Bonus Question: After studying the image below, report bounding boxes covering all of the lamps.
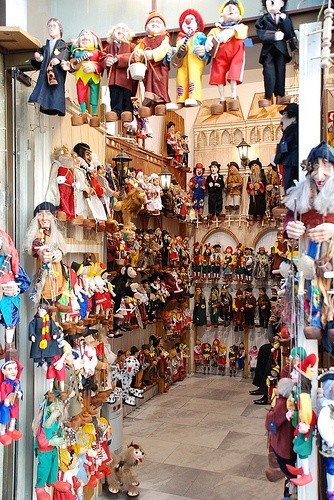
[112,149,133,200]
[158,165,173,217]
[237,137,251,168]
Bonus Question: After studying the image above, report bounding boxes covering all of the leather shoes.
[249,387,263,395]
[253,395,268,405]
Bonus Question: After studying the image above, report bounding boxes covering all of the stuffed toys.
[0,1,334,500]
